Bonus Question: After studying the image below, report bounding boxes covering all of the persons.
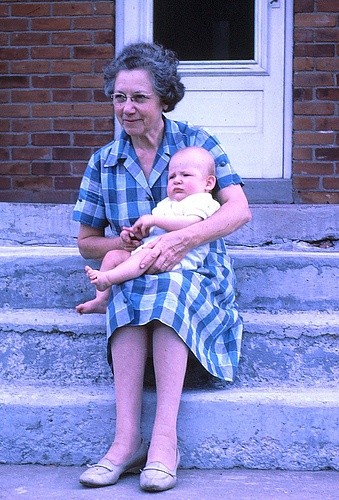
[75,145,222,317]
[69,40,255,495]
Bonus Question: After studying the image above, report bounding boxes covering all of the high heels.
[139,448,180,492]
[78,437,148,486]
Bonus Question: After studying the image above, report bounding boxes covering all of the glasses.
[110,92,156,103]
